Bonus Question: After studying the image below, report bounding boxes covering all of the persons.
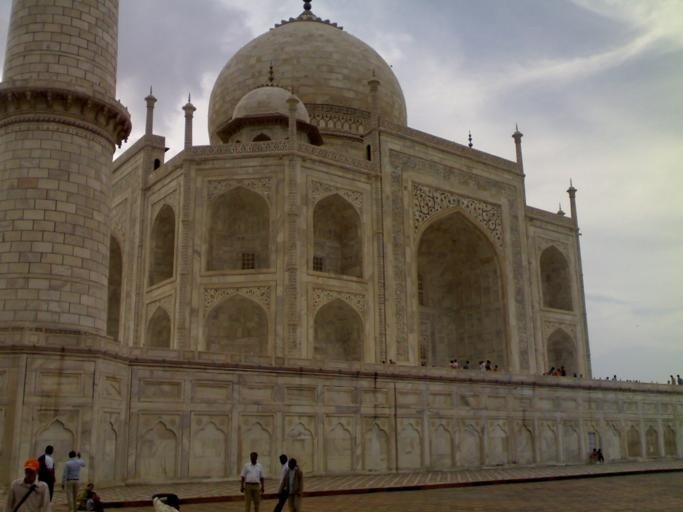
[37,445,56,503]
[667,375,681,385]
[239,452,265,512]
[607,375,617,381]
[547,366,583,378]
[272,454,289,512]
[75,483,96,512]
[151,493,181,512]
[590,448,598,462]
[450,360,499,371]
[597,448,604,464]
[85,492,104,512]
[382,360,396,364]
[60,449,86,512]
[5,458,51,512]
[279,457,304,512]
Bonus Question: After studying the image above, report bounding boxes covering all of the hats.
[23,458,40,471]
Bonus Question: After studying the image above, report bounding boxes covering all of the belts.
[246,482,259,485]
[69,479,79,480]
